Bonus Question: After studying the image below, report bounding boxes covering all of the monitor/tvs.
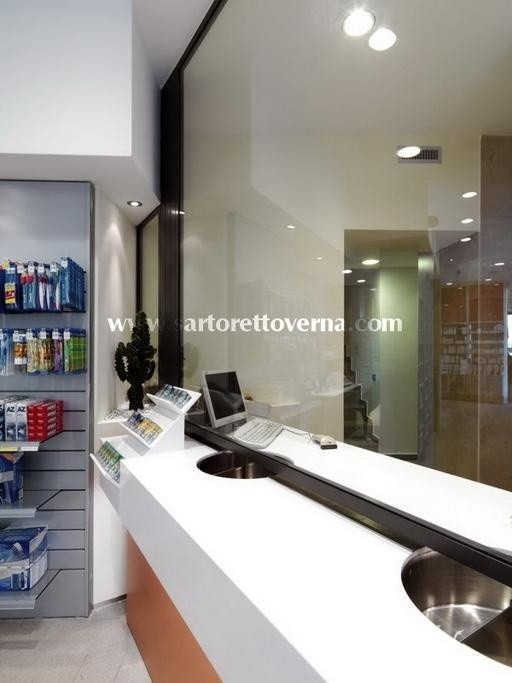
[199,368,248,437]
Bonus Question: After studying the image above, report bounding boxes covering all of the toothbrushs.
[1,259,85,375]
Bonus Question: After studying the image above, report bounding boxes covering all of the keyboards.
[234,416,284,449]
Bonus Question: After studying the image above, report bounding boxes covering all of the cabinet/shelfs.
[0,175,103,619]
[87,381,203,488]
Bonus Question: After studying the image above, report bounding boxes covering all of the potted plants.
[113,306,159,413]
